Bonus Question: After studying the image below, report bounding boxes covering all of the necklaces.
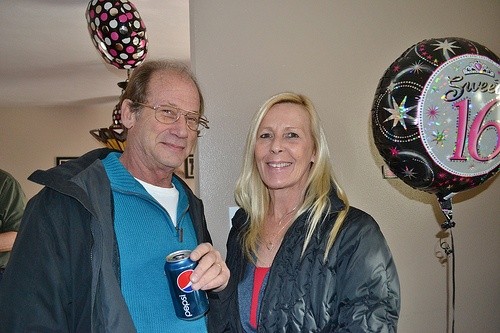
[265,217,293,251]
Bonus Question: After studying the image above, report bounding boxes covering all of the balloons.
[370,37,500,222]
[83,0,149,70]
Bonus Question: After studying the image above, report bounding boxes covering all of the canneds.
[164,249,210,321]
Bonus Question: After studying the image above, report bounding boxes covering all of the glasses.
[135,101,209,131]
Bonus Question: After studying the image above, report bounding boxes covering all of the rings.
[216,263,222,269]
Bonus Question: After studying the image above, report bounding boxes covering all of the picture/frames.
[184,154,194,178]
[56,157,80,166]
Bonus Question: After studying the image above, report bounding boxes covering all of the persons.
[226,92,401,333]
[0,57,238,333]
[0,168,30,272]
